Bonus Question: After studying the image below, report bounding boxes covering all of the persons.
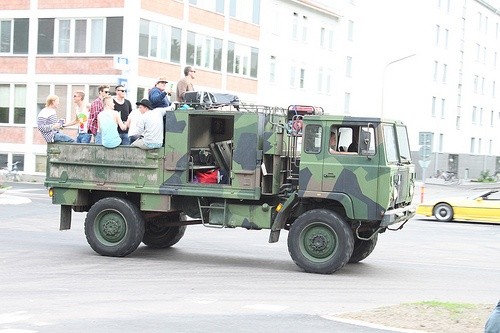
[87,84,111,144]
[127,98,179,150]
[60,91,92,143]
[113,85,133,145]
[97,97,128,149]
[148,78,171,147]
[176,65,196,102]
[329,132,359,155]
[37,95,75,143]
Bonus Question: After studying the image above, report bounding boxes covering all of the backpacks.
[199,149,215,166]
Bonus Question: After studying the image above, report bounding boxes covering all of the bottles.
[170,83,176,101]
[184,100,187,105]
[190,99,193,106]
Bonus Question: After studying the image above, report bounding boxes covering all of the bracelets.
[63,124,65,127]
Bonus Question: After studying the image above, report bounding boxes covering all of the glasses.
[74,95,80,97]
[105,91,110,94]
[190,70,196,73]
[118,90,127,92]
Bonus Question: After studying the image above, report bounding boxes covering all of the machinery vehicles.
[43,89,417,274]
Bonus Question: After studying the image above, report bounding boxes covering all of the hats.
[136,99,154,110]
[155,77,168,84]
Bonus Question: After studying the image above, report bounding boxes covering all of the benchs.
[188,147,216,183]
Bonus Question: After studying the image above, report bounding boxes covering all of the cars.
[418,186,500,224]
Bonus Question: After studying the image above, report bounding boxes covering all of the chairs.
[340,146,345,152]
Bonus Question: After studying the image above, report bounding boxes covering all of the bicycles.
[0,161,24,183]
